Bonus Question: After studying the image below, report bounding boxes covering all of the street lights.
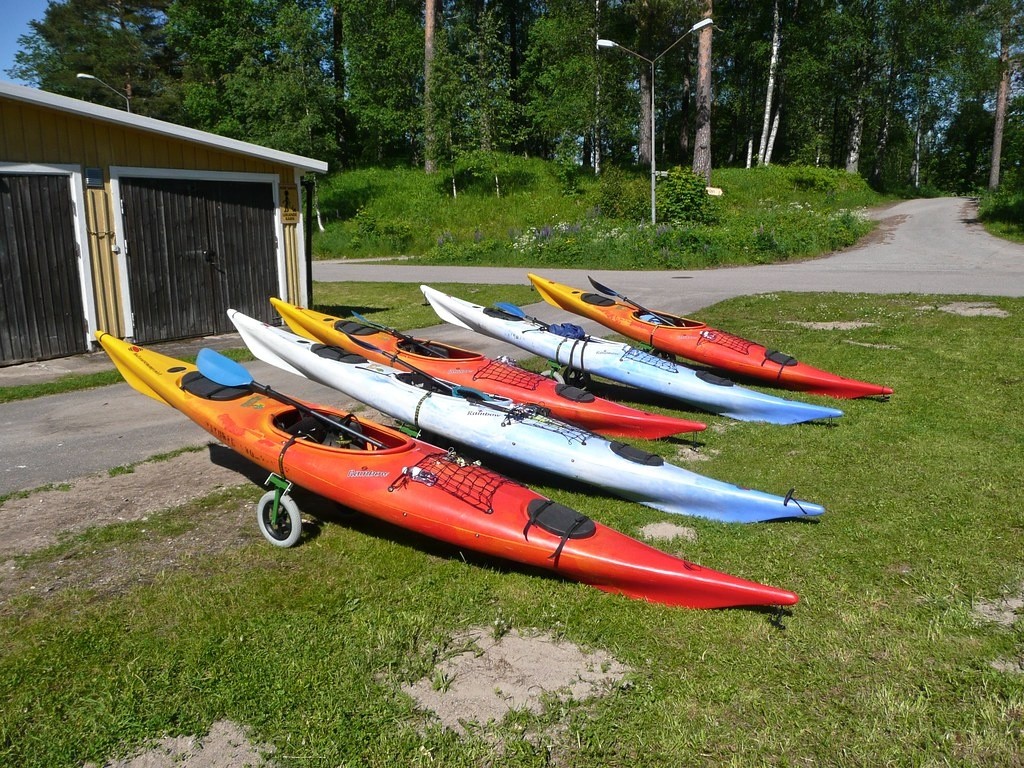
[76,72,129,113]
[597,18,713,226]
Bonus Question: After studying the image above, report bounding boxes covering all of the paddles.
[351,310,448,359]
[196,348,389,449]
[338,325,493,400]
[587,275,676,327]
[494,301,549,329]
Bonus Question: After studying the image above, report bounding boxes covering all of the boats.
[227,298,827,522]
[92,330,799,611]
[420,274,894,424]
